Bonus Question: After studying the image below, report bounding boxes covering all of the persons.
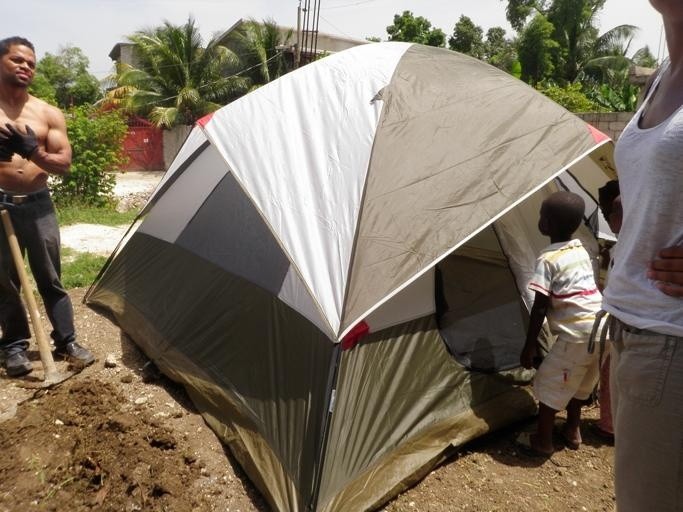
[518,180,624,455]
[0,36,95,378]
[608,0,682,512]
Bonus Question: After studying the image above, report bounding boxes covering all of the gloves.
[0,123,39,162]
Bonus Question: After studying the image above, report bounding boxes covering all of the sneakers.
[55,343,95,364]
[5,352,33,375]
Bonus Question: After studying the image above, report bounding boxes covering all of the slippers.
[553,419,578,450]
[511,431,553,457]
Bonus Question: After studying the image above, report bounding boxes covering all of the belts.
[0,189,50,206]
